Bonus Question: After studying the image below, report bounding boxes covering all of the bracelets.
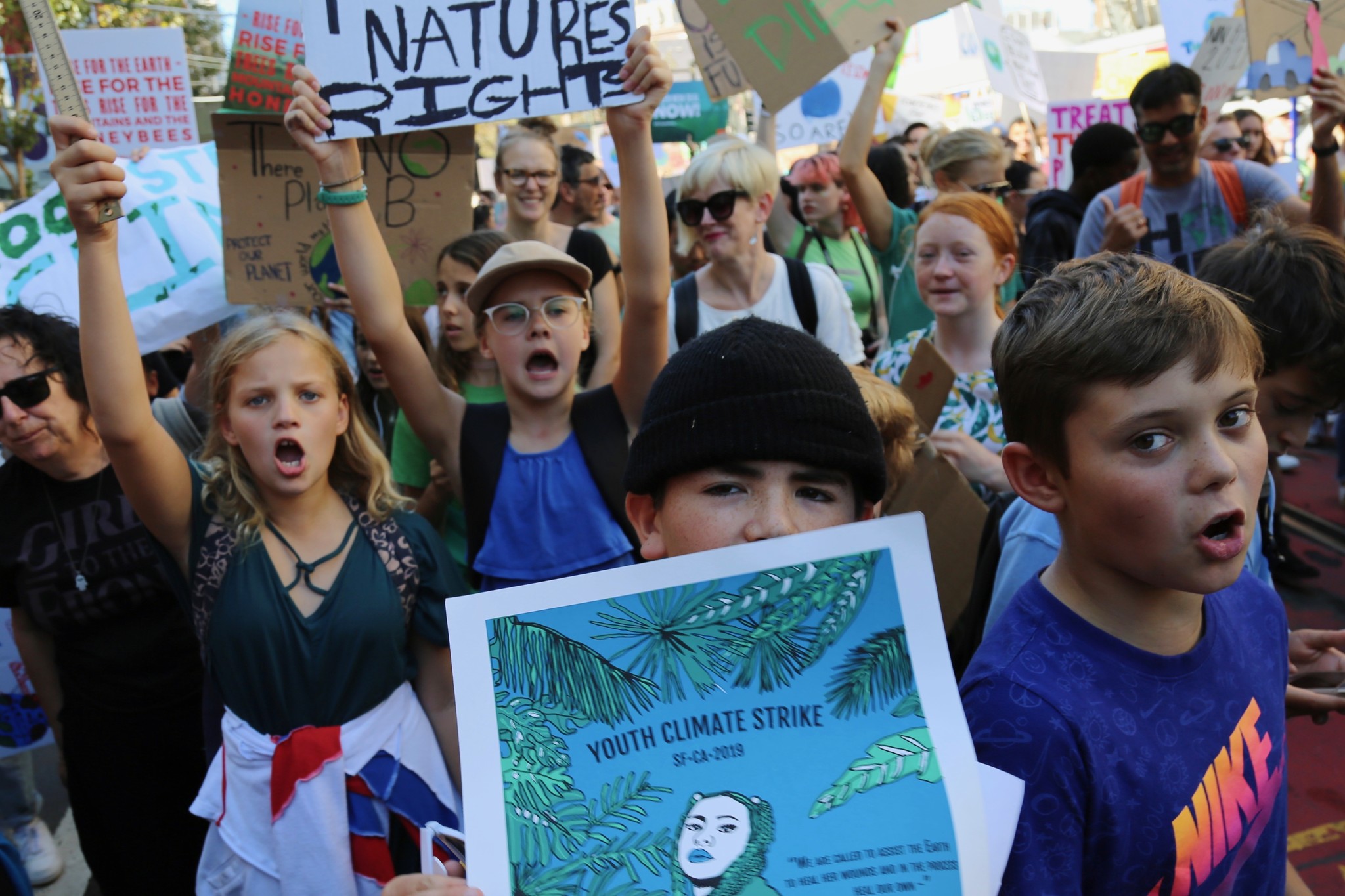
[316,170,366,187]
[316,186,369,204]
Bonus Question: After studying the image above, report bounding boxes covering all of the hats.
[623,317,890,506]
[463,239,593,315]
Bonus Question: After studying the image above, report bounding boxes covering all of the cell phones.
[1287,671,1344,696]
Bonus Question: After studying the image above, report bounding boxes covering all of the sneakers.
[12,820,64,886]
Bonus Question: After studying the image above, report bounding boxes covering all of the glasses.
[676,187,750,226]
[578,176,600,186]
[975,180,1013,196]
[1212,135,1253,153]
[0,367,59,417]
[1135,103,1200,143]
[480,296,587,337]
[502,168,559,187]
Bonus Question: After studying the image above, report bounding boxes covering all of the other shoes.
[1256,494,1323,577]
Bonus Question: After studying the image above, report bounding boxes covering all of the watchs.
[1311,134,1339,157]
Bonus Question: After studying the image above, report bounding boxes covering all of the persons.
[872,191,1022,501]
[0,19,1345,896]
[390,230,582,581]
[666,137,866,369]
[491,126,623,393]
